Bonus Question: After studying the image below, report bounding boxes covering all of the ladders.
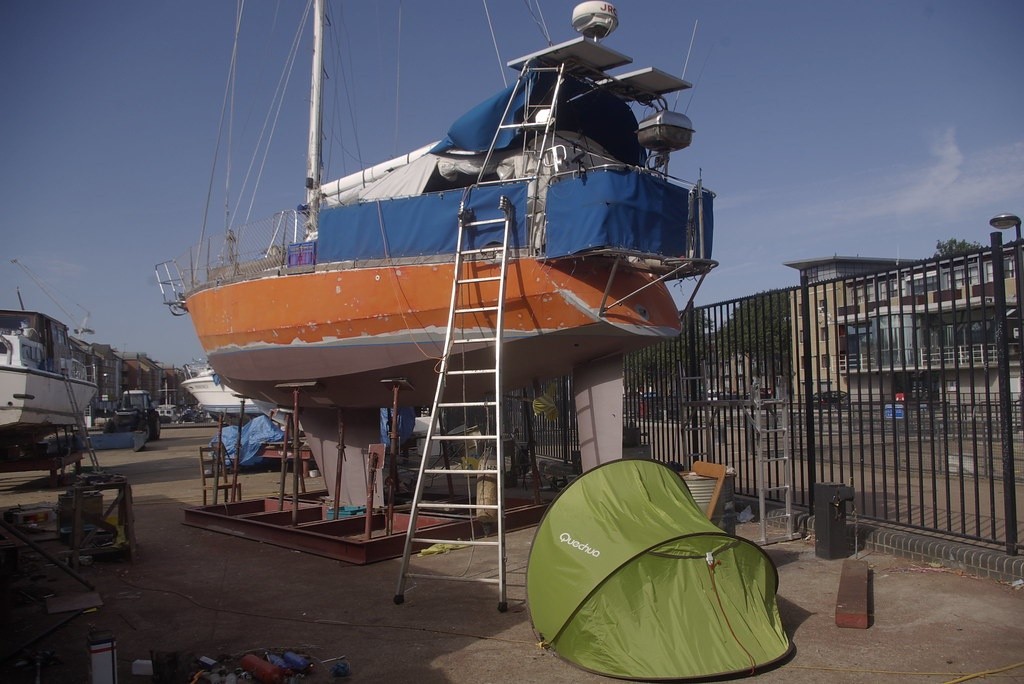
[392,195,512,614]
[61,373,101,473]
[677,360,802,546]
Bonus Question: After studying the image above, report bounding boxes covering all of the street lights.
[988,211,1024,430]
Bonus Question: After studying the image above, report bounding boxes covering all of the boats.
[0,322,101,433]
[179,353,266,416]
[148,1,722,416]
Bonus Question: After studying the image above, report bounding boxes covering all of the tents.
[524,457,793,682]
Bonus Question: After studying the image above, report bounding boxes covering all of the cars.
[812,389,849,411]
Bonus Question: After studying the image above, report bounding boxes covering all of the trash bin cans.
[814,482,848,559]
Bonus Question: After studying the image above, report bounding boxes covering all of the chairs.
[199,443,241,505]
